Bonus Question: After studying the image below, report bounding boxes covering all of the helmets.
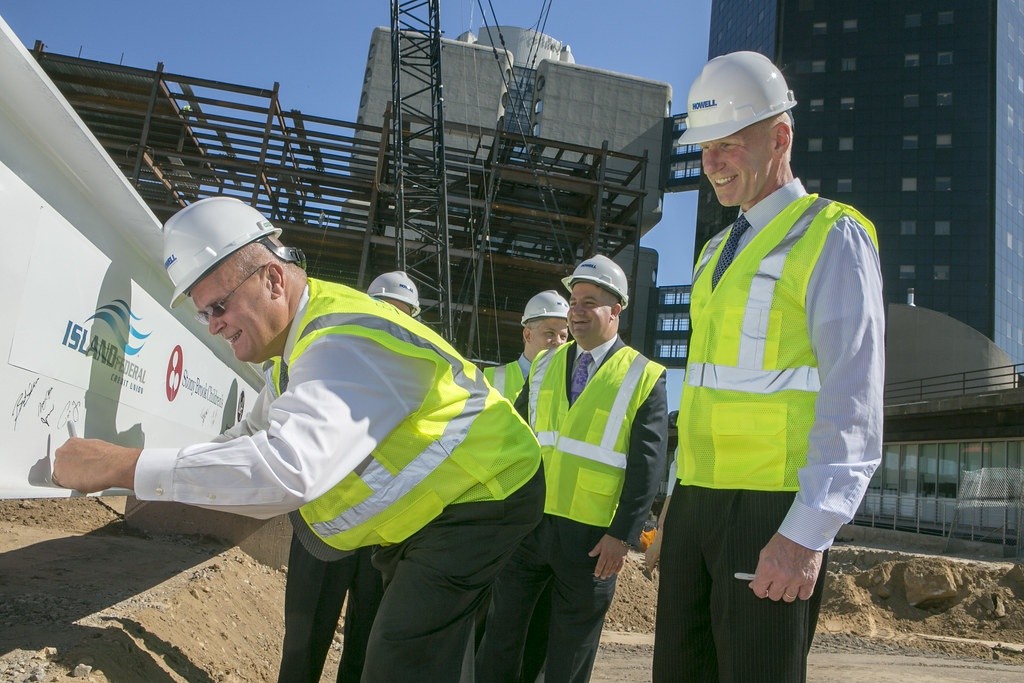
[678,51,798,145]
[561,254,629,311]
[366,270,421,318]
[162,196,306,301]
[521,290,570,326]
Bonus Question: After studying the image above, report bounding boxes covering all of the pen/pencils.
[734,572,755,581]
[67,420,77,439]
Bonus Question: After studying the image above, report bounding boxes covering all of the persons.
[475,254,669,683]
[51,197,547,682]
[479,290,569,407]
[651,50,887,683]
[277,268,421,683]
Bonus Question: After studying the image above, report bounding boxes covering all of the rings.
[785,593,797,598]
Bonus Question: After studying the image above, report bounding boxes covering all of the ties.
[571,354,593,408]
[711,211,751,292]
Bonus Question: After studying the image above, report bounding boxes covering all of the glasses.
[195,266,266,324]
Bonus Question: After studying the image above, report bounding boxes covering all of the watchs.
[621,540,632,547]
